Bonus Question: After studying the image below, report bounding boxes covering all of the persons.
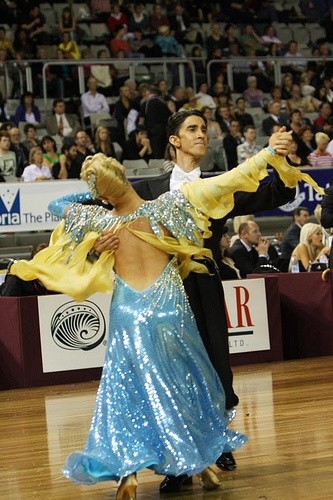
[310,193,333,271]
[51,140,81,179]
[94,126,117,159]
[230,219,277,275]
[0,0,333,181]
[237,125,264,165]
[81,75,109,125]
[58,130,93,180]
[22,123,47,155]
[0,115,324,500]
[289,223,329,274]
[45,98,81,137]
[218,226,246,279]
[9,127,31,178]
[282,208,313,264]
[72,110,307,494]
[224,117,244,172]
[15,91,42,125]
[22,146,53,180]
[89,50,117,97]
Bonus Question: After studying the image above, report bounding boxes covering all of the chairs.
[40,3,325,56]
[5,98,319,175]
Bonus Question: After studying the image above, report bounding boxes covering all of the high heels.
[199,466,222,490]
[116,471,138,500]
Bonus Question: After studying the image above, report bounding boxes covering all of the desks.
[0,271,333,391]
[0,166,333,234]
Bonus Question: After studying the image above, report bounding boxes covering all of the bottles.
[291,255,300,274]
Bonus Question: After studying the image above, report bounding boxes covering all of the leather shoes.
[160,474,192,493]
[215,452,237,471]
